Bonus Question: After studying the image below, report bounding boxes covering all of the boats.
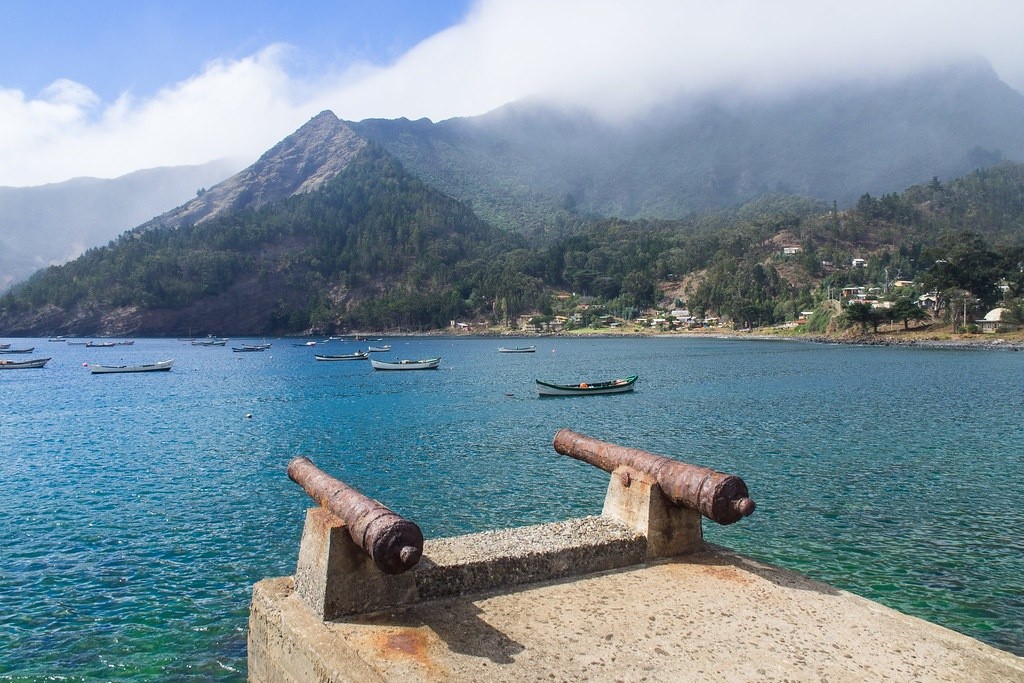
[0,347,36,354]
[48,338,66,342]
[0,343,11,349]
[314,351,370,361]
[368,345,391,353]
[109,341,135,345]
[191,333,230,347]
[0,358,52,369]
[498,344,536,354]
[536,374,639,397]
[82,358,176,374]
[231,343,274,352]
[316,336,384,346]
[177,337,197,340]
[292,341,317,347]
[371,357,441,370]
[85,342,115,347]
